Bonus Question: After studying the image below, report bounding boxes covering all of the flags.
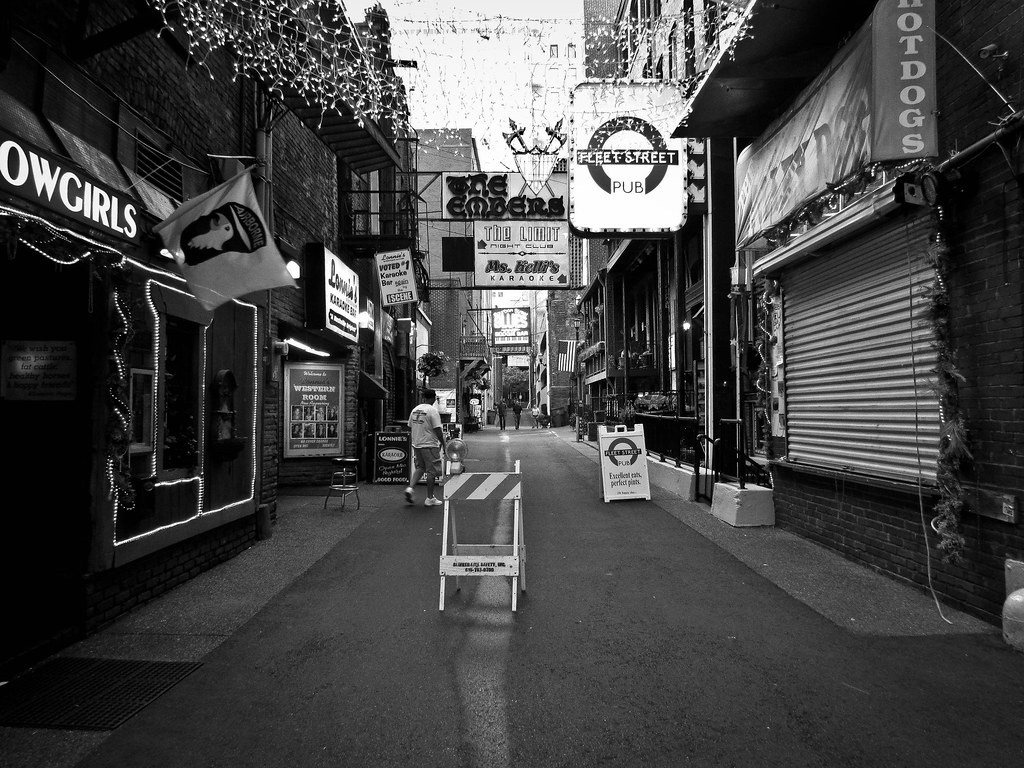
[557,340,576,373]
[156,171,296,310]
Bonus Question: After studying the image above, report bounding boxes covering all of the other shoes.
[501,428,502,430]
[515,428,518,430]
[504,427,505,430]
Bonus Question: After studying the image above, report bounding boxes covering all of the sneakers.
[424,495,443,506]
[404,486,416,504]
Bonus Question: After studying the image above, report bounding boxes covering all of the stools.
[324,457,360,512]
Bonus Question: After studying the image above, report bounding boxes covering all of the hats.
[423,389,436,398]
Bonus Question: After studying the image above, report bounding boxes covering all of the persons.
[512,401,523,430]
[404,388,447,507]
[532,404,540,429]
[497,399,507,430]
[292,406,338,438]
